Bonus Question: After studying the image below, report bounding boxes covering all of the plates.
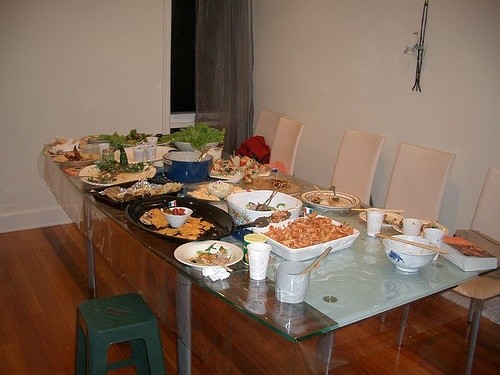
[174,240,244,268]
[266,248,357,282]
[125,195,236,244]
[44,141,305,210]
[252,215,360,262]
[300,190,450,237]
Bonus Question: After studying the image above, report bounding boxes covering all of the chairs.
[250,110,500,375]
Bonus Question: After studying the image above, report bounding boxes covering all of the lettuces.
[169,122,226,149]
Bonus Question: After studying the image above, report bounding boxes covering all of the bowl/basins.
[171,138,219,152]
[382,235,438,273]
[163,150,213,183]
[160,207,193,228]
[381,270,433,302]
[227,190,303,232]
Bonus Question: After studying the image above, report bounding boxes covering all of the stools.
[75,292,165,375]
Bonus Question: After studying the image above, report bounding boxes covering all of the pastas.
[264,211,353,249]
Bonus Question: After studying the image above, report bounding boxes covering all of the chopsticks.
[375,233,448,255]
[350,208,405,212]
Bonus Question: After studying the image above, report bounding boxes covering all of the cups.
[272,261,311,304]
[272,299,305,334]
[246,242,272,281]
[246,278,268,304]
[242,234,268,268]
[80,144,98,155]
[361,235,380,265]
[134,136,158,162]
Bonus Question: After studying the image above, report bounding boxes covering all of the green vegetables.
[90,128,168,173]
[205,242,227,255]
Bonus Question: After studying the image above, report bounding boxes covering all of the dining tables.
[37,143,500,375]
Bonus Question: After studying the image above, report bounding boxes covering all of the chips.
[146,209,213,240]
